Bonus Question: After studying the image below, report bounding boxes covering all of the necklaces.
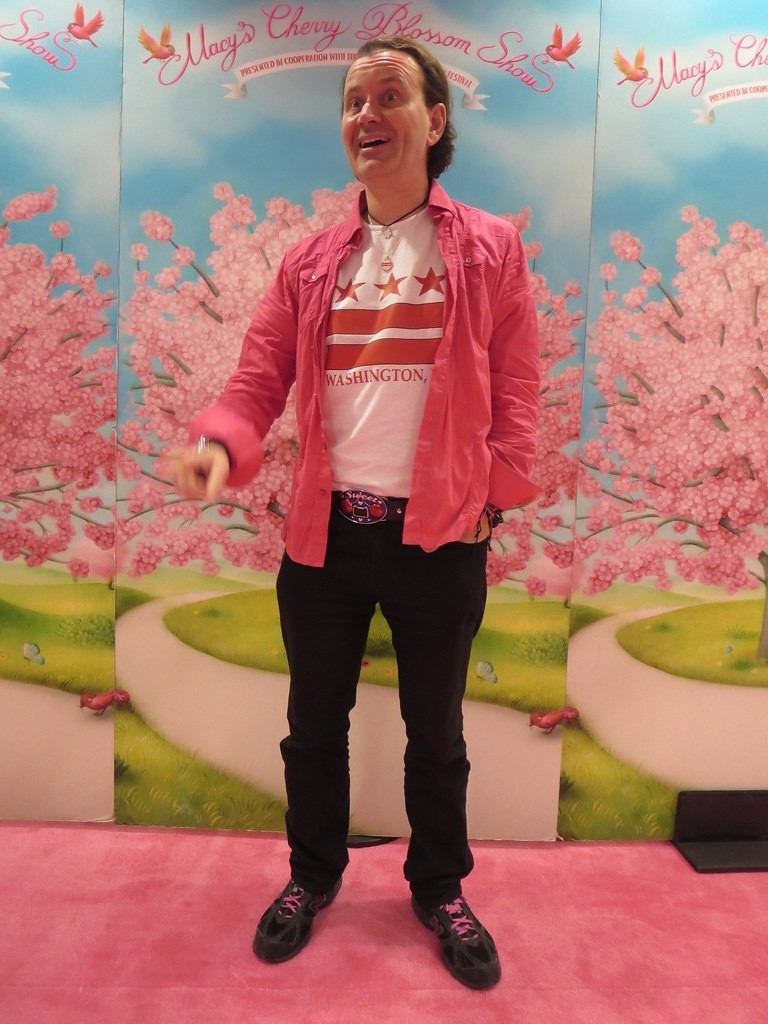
[368,198,428,238]
[368,213,418,270]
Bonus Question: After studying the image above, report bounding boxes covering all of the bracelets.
[197,435,231,466]
[484,503,503,527]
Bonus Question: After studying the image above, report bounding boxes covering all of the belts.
[330,488,407,526]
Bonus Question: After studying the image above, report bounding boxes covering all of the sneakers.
[411,894,500,991]
[253,872,343,964]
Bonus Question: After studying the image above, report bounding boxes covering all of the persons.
[174,37,539,991]
